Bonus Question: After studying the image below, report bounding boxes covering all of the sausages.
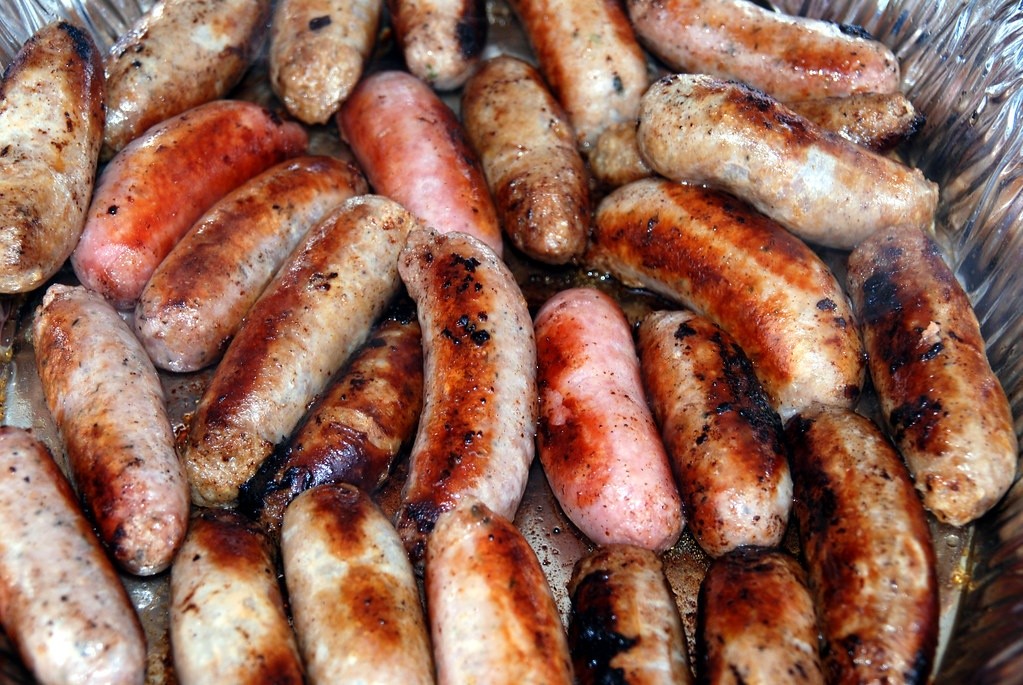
[0,0,1023,685]
